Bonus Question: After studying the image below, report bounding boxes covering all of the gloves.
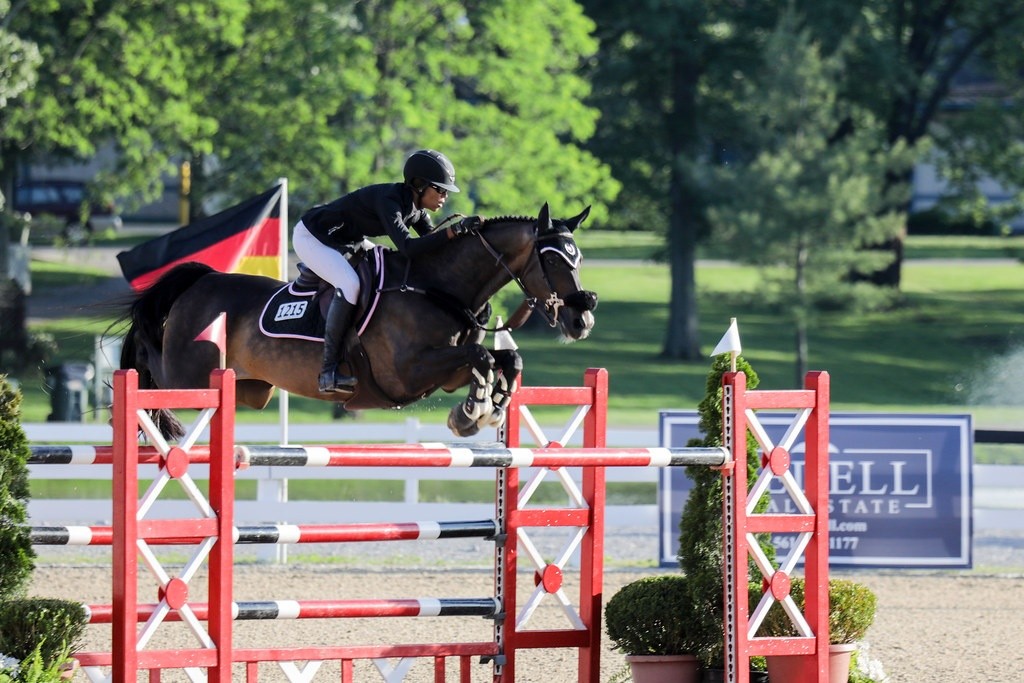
[451,216,481,236]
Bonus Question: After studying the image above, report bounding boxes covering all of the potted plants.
[679,347,780,683]
[606,577,722,682]
[742,579,876,683]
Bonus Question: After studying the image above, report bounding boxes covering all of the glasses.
[428,183,447,194]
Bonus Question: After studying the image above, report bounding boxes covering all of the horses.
[106,199,598,445]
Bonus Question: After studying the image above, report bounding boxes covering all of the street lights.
[789,112,861,394]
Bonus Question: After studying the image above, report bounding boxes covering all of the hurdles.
[18,366,833,682]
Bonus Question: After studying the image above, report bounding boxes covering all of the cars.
[10,177,125,248]
[915,160,1024,242]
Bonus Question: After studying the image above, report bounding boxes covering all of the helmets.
[404,149,460,193]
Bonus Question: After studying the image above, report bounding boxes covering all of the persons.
[292,148,460,395]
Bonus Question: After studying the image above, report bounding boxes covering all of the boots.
[317,288,358,392]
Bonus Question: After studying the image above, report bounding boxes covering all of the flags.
[116,183,281,289]
